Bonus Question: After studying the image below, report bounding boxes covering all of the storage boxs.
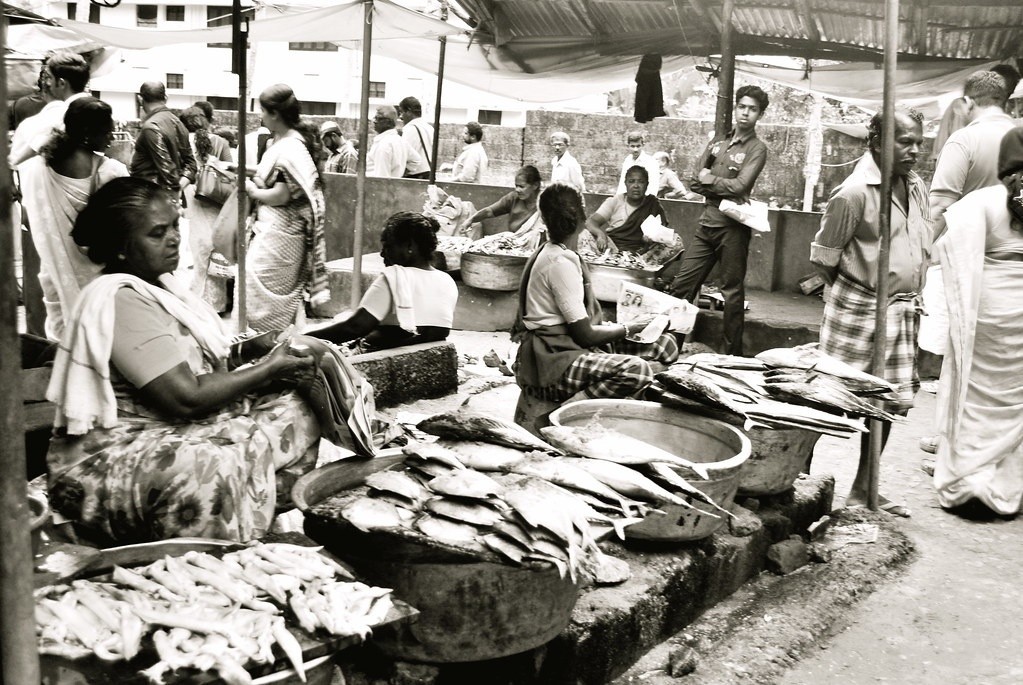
[798,272,824,296]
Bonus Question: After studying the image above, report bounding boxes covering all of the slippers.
[846,499,907,517]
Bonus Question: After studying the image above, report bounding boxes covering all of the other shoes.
[920,434,938,453]
[920,458,935,475]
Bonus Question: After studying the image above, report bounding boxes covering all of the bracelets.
[228,347,237,368]
[619,322,629,339]
[237,343,244,364]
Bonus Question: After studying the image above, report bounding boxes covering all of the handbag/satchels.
[193,135,236,205]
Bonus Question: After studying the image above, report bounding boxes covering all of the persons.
[299,209,460,352]
[47,175,328,545]
[621,292,642,309]
[668,85,770,356]
[5,47,434,346]
[576,166,685,272]
[458,165,550,252]
[448,122,488,186]
[811,110,933,518]
[508,184,654,437]
[914,65,1022,523]
[653,151,686,200]
[548,131,586,208]
[615,132,660,198]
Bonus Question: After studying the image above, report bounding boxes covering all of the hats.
[318,122,343,139]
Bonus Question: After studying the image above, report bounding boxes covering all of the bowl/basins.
[461,252,528,290]
[589,264,656,302]
[548,399,753,541]
[291,454,579,662]
[736,426,822,494]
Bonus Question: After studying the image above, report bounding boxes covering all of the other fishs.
[342,412,738,584]
[655,345,920,433]
